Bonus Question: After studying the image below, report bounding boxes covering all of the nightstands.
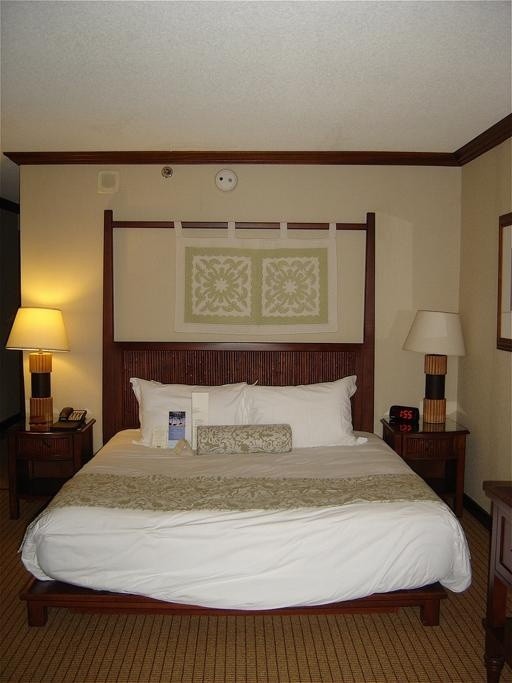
[380,417,470,518]
[3,418,96,519]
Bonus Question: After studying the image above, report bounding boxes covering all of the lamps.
[5,306,71,424]
[402,309,466,424]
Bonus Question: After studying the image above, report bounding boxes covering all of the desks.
[481,480,511,683]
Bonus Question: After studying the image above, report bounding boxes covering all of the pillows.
[239,375,368,449]
[129,377,247,450]
[197,424,292,455]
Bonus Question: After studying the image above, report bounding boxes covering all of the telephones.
[59,408,87,422]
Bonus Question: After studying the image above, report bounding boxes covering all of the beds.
[17,210,473,628]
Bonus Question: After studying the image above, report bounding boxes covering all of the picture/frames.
[496,211,512,351]
